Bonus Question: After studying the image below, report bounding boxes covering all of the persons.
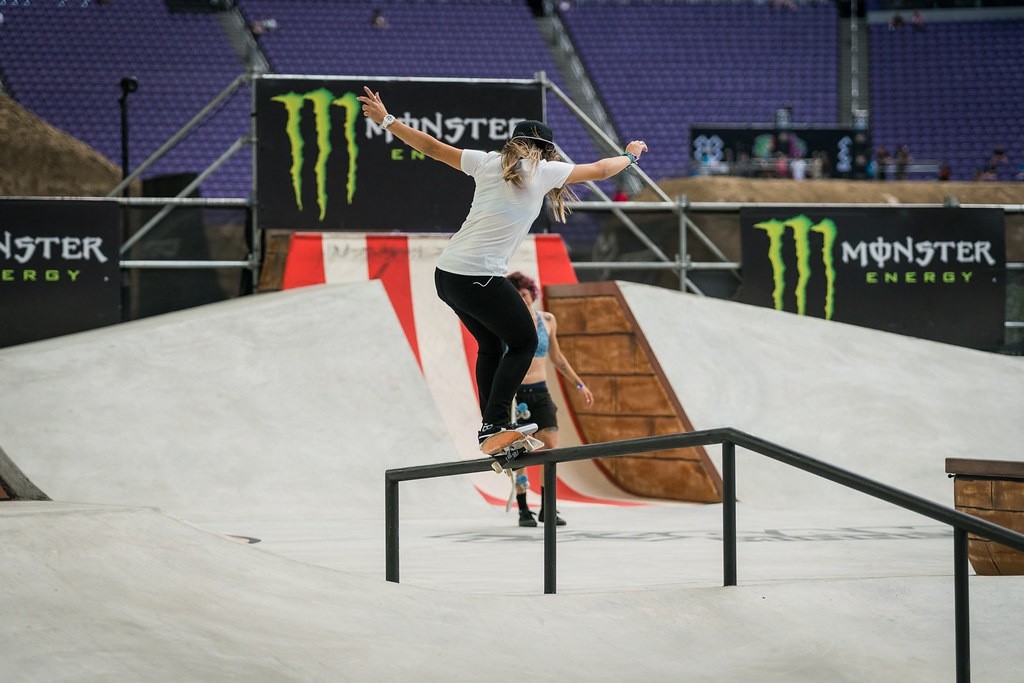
[732,132,1009,181]
[505,270,593,527]
[357,85,649,455]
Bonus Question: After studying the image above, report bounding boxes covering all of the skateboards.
[481,432,546,473]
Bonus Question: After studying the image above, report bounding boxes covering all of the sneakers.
[519,508,537,527]
[538,508,566,526]
[478,423,539,449]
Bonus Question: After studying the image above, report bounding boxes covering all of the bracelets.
[575,383,585,391]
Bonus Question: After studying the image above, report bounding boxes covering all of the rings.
[370,95,376,100]
[640,141,644,145]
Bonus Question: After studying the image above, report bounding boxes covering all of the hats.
[512,120,556,153]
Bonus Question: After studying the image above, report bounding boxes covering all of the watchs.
[381,115,395,129]
[622,152,638,171]
